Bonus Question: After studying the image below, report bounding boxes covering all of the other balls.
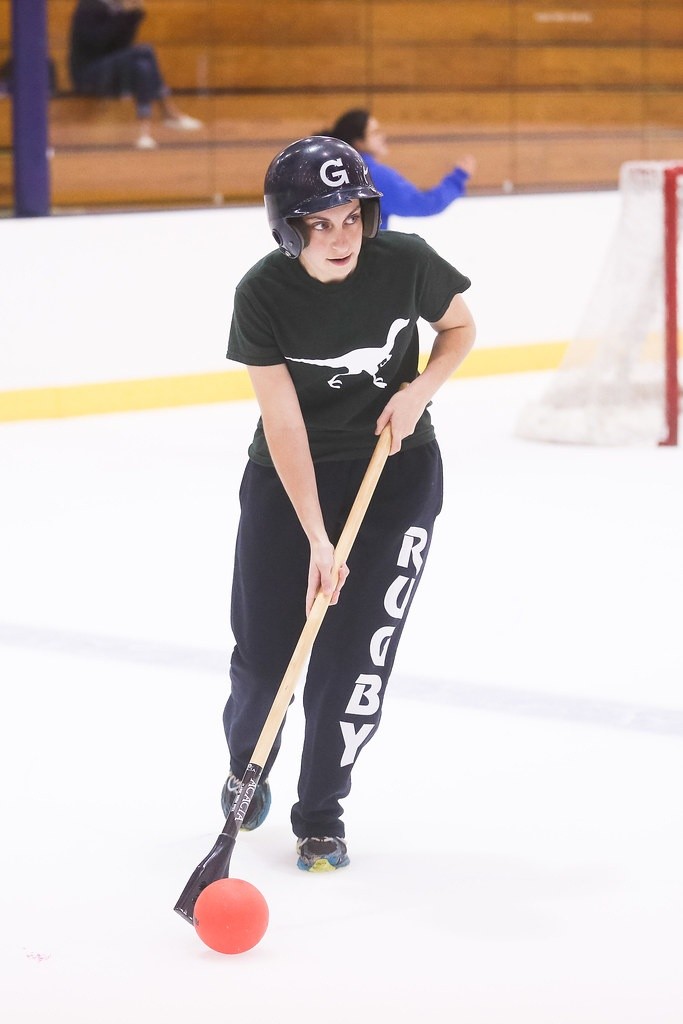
[195,878,271,953]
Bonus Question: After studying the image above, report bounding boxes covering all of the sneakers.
[222,769,271,832]
[295,837,351,873]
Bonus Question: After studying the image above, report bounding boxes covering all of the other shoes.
[165,117,202,131]
[137,135,154,151]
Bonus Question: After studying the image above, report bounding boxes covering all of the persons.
[223,135,474,874]
[67,0,204,154]
[312,105,475,233]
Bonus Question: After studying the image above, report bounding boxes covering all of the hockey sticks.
[177,382,412,923]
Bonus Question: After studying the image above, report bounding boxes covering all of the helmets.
[263,136,384,259]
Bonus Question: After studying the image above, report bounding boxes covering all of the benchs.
[0,1,681,218]
